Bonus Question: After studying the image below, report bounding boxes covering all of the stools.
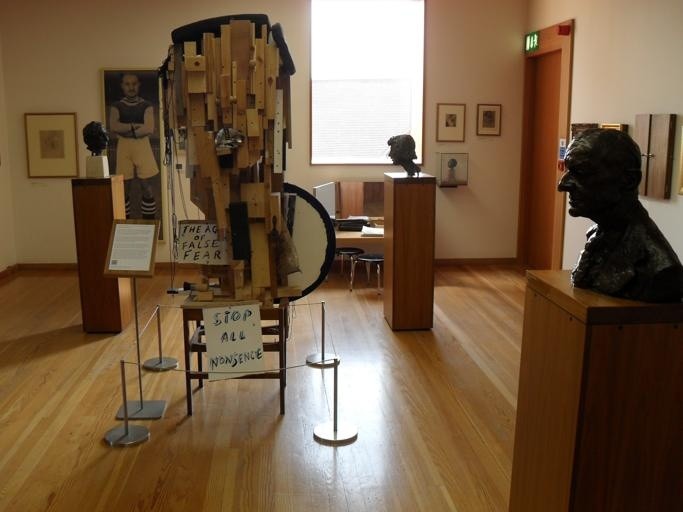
[325,248,384,295]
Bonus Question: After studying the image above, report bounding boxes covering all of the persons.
[109,74,161,219]
[387,134,421,177]
[558,127,683,303]
[83,121,109,155]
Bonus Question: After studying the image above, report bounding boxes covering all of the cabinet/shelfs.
[634,114,676,199]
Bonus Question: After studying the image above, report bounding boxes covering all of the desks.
[326,223,384,282]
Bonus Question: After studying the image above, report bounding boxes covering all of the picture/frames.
[437,103,465,142]
[572,123,628,141]
[476,104,501,136]
[102,68,169,243]
[24,113,79,178]
[676,126,683,195]
